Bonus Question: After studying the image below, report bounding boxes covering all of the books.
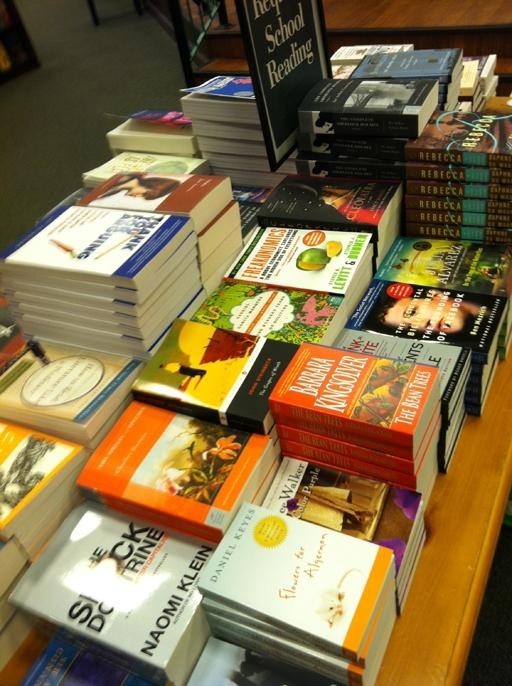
[0,43,512,686]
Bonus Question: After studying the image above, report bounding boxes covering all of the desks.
[0,338,512,686]
